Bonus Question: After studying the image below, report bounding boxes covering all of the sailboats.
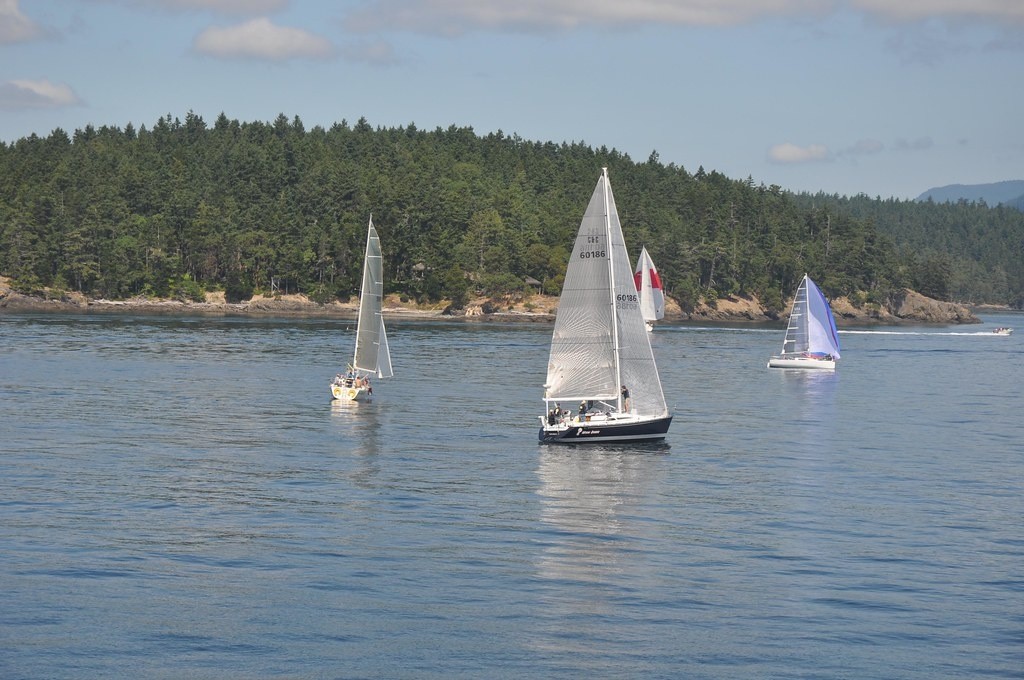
[328,213,394,402]
[536,166,675,443]
[766,273,840,370]
[632,245,666,332]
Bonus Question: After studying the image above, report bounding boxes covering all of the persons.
[542,384,632,427]
[334,373,375,396]
